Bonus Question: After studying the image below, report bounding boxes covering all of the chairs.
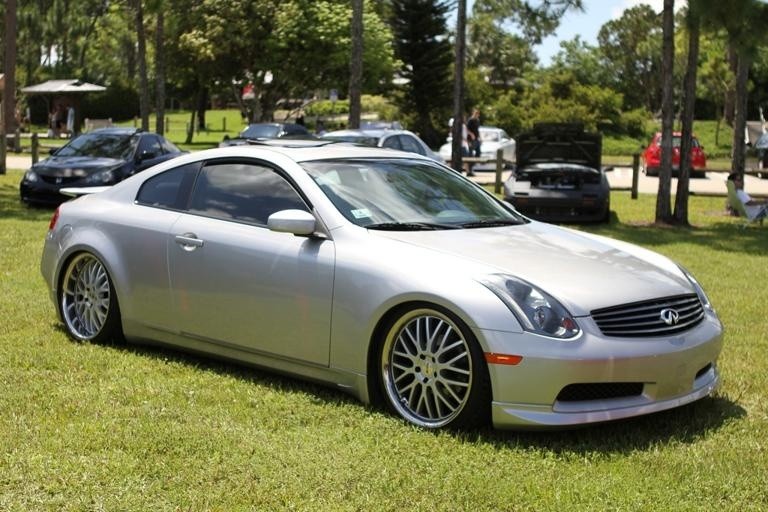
[724,178,768,233]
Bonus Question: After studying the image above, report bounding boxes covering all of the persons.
[727,173,767,221]
[46,108,57,138]
[51,104,62,139]
[459,114,476,173]
[465,108,481,176]
[65,104,74,139]
